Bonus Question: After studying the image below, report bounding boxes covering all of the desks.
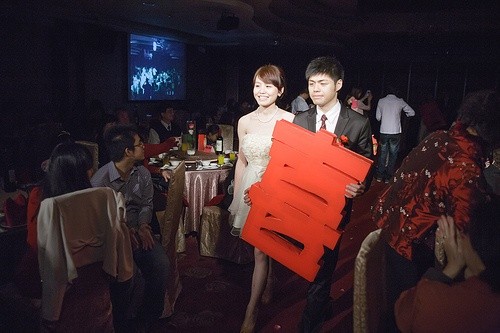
[160,154,234,233]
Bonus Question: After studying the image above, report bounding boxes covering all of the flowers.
[186,122,196,142]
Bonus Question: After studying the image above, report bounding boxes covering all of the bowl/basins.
[201,160,210,165]
[171,161,179,166]
[150,158,155,162]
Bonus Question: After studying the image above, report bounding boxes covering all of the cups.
[217,154,224,165]
[230,151,235,161]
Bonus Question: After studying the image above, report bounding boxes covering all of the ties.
[320,115,327,131]
[168,126,170,131]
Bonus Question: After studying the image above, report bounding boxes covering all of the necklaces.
[256,105,278,122]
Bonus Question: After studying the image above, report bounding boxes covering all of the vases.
[187,130,196,155]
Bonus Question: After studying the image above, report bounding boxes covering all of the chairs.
[0,122,253,332]
[351,227,390,332]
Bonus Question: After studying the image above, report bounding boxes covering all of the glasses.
[129,142,145,149]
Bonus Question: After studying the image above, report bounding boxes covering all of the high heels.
[238,306,259,333]
[261,276,279,304]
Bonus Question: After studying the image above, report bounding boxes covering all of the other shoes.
[376,178,382,182]
[384,180,390,184]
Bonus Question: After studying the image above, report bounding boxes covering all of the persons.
[277,87,500,194]
[90,124,176,333]
[395,195,500,333]
[86,98,257,208]
[227,65,295,333]
[245,55,375,333]
[371,87,500,333]
[18,142,92,281]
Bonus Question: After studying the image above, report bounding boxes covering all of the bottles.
[215,129,223,155]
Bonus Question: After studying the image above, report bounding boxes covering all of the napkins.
[3,193,26,225]
